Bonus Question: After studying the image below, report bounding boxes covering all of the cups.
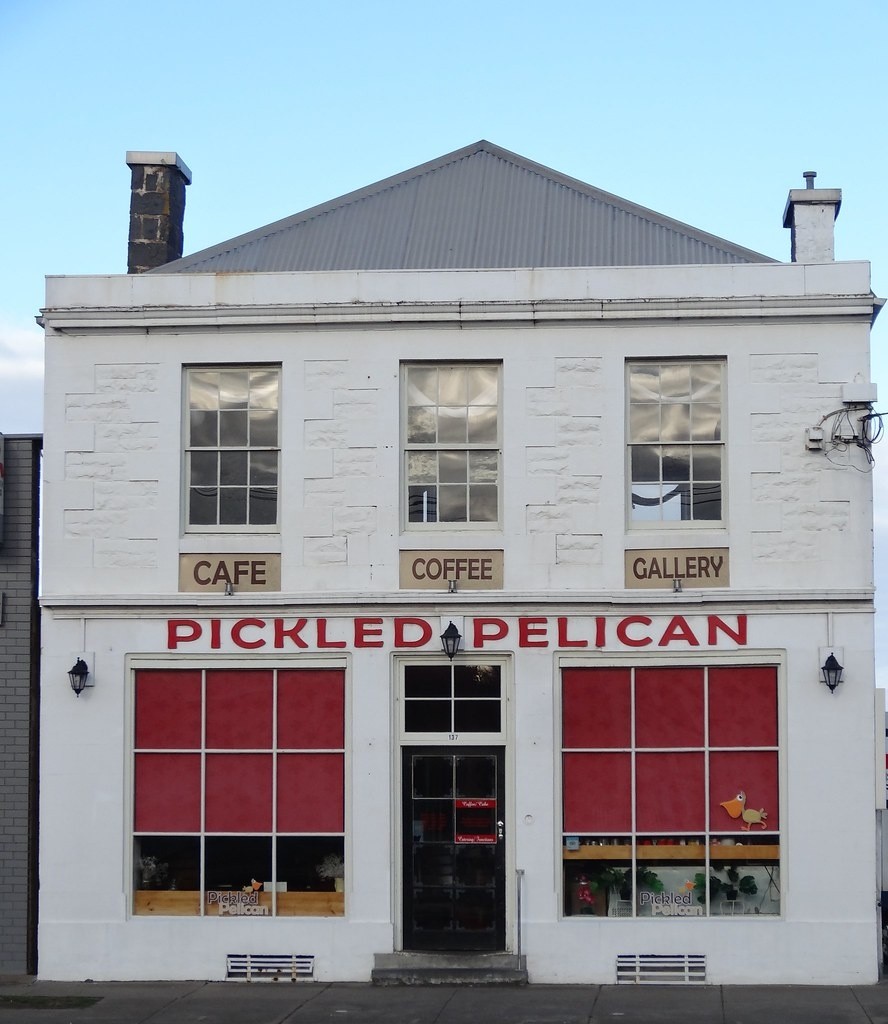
[727,890,738,899]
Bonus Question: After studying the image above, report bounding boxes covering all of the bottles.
[170,878,177,890]
[620,879,632,900]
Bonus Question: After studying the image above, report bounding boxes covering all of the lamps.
[441,621,462,660]
[69,657,89,698]
[821,652,844,692]
